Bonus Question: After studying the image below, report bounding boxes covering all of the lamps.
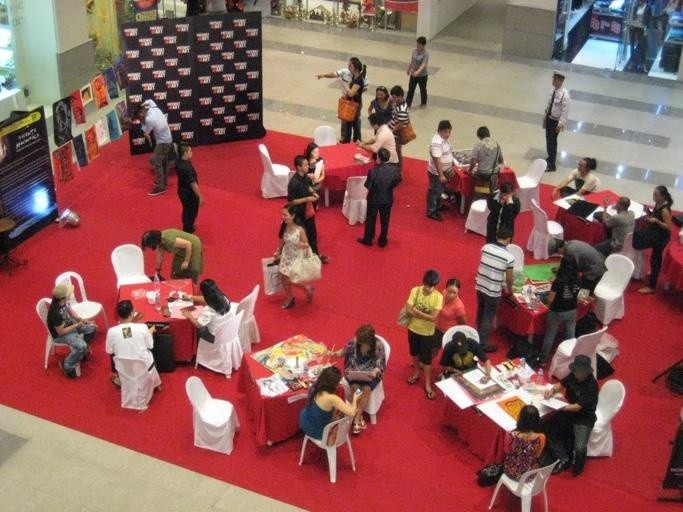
[53,207,80,229]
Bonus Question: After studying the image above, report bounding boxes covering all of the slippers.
[353,418,368,434]
[407,376,419,385]
[424,387,435,400]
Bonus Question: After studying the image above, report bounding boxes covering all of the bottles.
[520,358,544,385]
[523,284,542,312]
[152,271,162,311]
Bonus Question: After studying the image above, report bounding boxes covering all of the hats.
[453,331,468,354]
[51,284,74,300]
[552,71,564,81]
[547,238,556,255]
[569,354,594,374]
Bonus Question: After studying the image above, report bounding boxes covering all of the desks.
[435,354,569,466]
[497,258,596,345]
[237,332,346,447]
[442,162,520,214]
[553,188,653,252]
[655,228,683,291]
[116,277,199,364]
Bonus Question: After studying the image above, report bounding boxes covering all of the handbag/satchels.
[477,462,503,487]
[328,413,345,446]
[445,168,456,180]
[398,122,416,145]
[508,340,546,368]
[490,175,498,195]
[396,305,413,329]
[632,230,654,249]
[290,254,322,283]
[575,312,603,337]
[338,99,359,123]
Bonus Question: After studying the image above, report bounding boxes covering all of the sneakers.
[483,344,497,352]
[419,104,426,109]
[638,287,656,294]
[152,180,169,187]
[148,187,164,195]
[59,361,77,378]
[320,255,329,263]
[111,374,120,389]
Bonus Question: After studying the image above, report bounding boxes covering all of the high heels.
[306,285,315,304]
[281,297,295,309]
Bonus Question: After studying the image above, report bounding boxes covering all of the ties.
[546,91,556,120]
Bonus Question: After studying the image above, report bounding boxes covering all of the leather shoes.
[573,462,584,476]
[429,204,450,220]
[552,462,569,475]
[357,238,363,243]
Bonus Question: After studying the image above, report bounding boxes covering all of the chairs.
[441,324,480,379]
[464,198,489,237]
[258,126,379,225]
[36,296,84,378]
[363,334,389,425]
[489,458,560,512]
[593,252,635,325]
[111,245,153,289]
[548,325,609,383]
[297,413,356,483]
[516,159,549,214]
[186,376,240,455]
[112,355,154,409]
[229,283,261,355]
[620,232,646,281]
[55,272,109,332]
[193,308,246,378]
[581,378,625,458]
[526,198,565,261]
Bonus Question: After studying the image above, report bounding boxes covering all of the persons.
[45,282,99,380]
[426,119,459,221]
[317,57,369,145]
[594,186,672,293]
[102,299,156,388]
[80,89,89,101]
[140,227,204,296]
[535,238,607,369]
[468,126,521,353]
[299,324,386,459]
[553,159,602,200]
[131,98,173,197]
[273,143,330,309]
[56,105,68,137]
[504,405,546,482]
[357,85,408,247]
[406,270,490,399]
[175,140,204,235]
[543,70,569,171]
[405,36,429,113]
[545,355,599,477]
[626,0,654,76]
[177,278,235,343]
[139,99,177,167]
